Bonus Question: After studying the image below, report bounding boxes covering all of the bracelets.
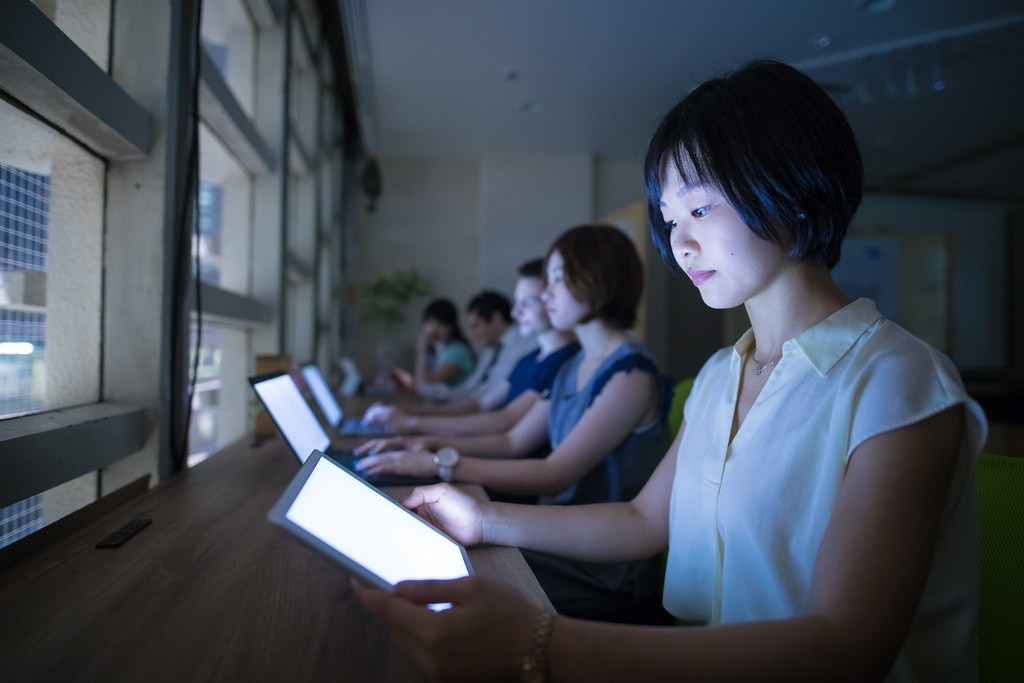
[521,607,558,683]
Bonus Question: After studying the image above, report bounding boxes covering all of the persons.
[353,57,986,683]
[355,223,671,626]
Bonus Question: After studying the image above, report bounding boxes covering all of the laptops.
[298,361,387,438]
[245,370,442,487]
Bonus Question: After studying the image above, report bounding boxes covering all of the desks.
[0,383,557,683]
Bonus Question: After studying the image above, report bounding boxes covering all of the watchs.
[434,446,460,483]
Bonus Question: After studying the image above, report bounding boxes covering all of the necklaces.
[753,347,783,375]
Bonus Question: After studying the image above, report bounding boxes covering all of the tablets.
[266,450,475,612]
